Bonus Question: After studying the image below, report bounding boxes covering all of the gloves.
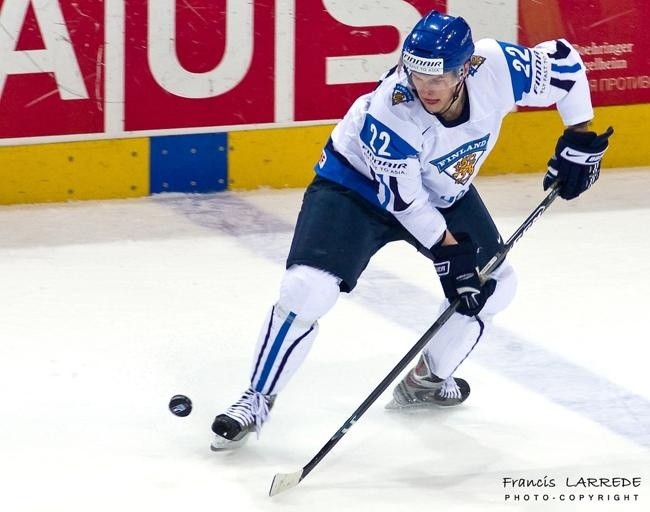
[434,231,496,317]
[543,127,615,200]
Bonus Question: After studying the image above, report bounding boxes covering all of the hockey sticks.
[270,180,560,495]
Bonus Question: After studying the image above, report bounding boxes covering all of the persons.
[210,7,614,455]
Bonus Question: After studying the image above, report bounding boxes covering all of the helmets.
[397,10,474,76]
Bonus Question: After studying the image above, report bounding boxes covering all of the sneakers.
[394,374,470,406]
[213,386,277,440]
